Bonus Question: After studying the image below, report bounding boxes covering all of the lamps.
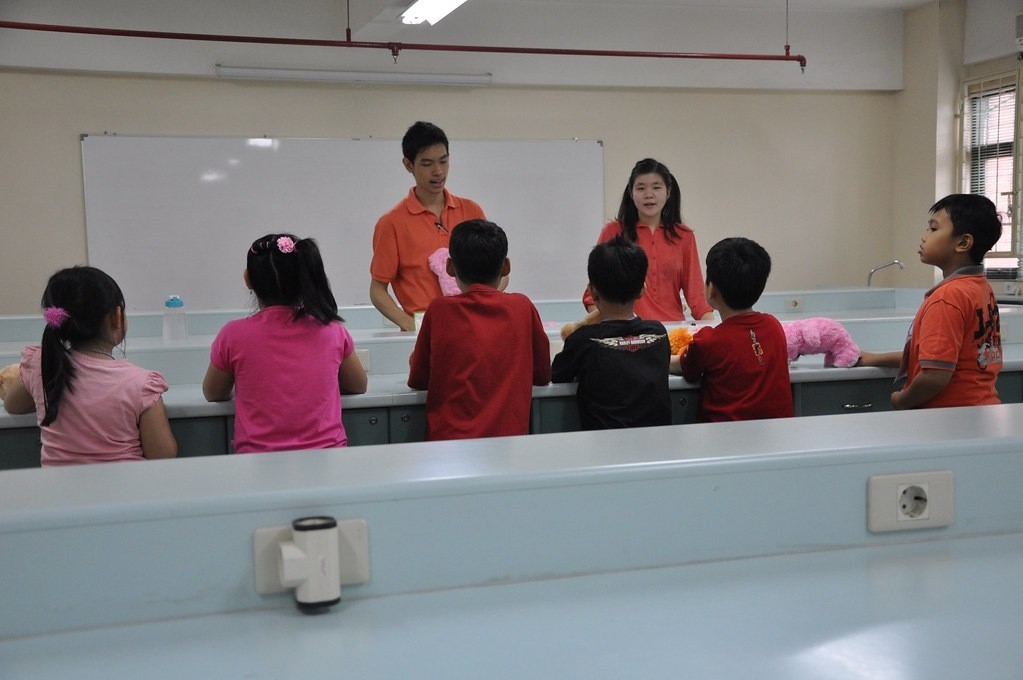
[393,0,468,26]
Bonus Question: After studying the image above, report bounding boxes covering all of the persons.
[551,233,672,430]
[369,121,509,333]
[582,158,716,321]
[200,234,368,454]
[669,236,793,422]
[407,219,550,441]
[853,193,1004,410]
[4,265,177,468]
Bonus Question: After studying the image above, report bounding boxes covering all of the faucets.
[866,259,905,287]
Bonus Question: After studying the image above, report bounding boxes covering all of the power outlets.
[254,519,368,595]
[866,470,954,530]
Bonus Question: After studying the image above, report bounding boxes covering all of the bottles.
[161,294,187,343]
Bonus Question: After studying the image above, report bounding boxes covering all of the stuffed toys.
[560,309,602,341]
[667,327,692,356]
[0,362,23,400]
[781,317,862,369]
[427,247,463,297]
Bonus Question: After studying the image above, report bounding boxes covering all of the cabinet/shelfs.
[0,378,903,471]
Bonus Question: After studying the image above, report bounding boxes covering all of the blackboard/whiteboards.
[79,134,605,338]
